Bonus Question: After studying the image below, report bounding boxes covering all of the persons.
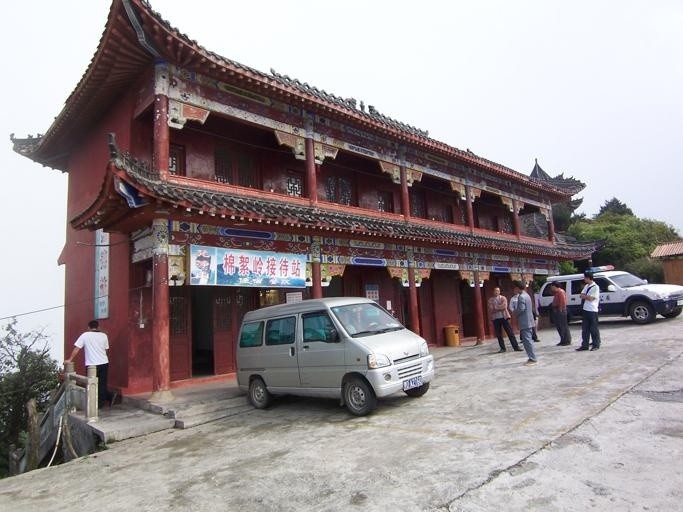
[62,319,119,409]
[486,268,604,368]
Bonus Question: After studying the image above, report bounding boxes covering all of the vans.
[234,295,436,418]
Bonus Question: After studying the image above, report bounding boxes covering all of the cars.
[537,263,682,324]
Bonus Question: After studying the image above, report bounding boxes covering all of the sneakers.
[524,359,538,366]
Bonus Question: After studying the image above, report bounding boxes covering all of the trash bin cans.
[443,325,460,347]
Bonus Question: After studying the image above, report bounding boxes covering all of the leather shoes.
[590,347,598,351]
[575,347,588,350]
